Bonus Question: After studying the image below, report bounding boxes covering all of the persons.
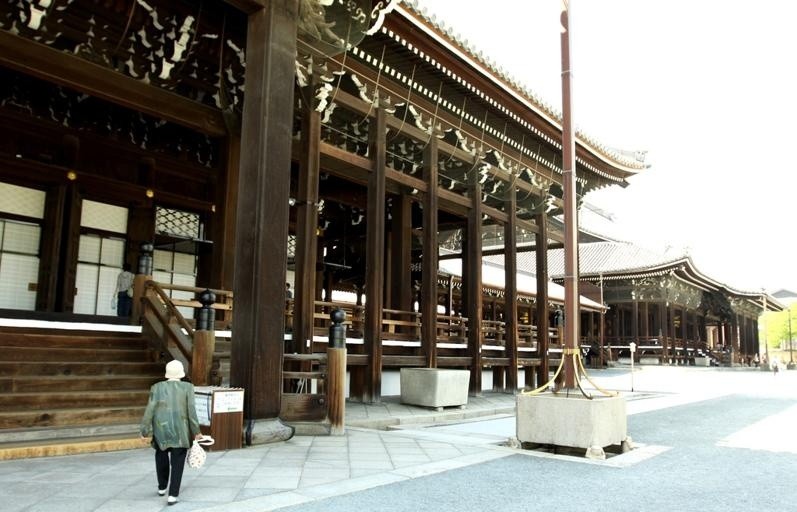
[714,342,779,376]
[286,283,292,298]
[137,360,202,503]
[114,262,135,317]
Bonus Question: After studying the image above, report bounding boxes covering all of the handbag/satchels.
[127,287,133,298]
[188,435,214,469]
[111,298,115,308]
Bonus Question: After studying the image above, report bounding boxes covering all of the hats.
[165,359,185,379]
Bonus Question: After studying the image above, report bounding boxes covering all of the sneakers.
[168,495,179,505]
[158,488,167,496]
